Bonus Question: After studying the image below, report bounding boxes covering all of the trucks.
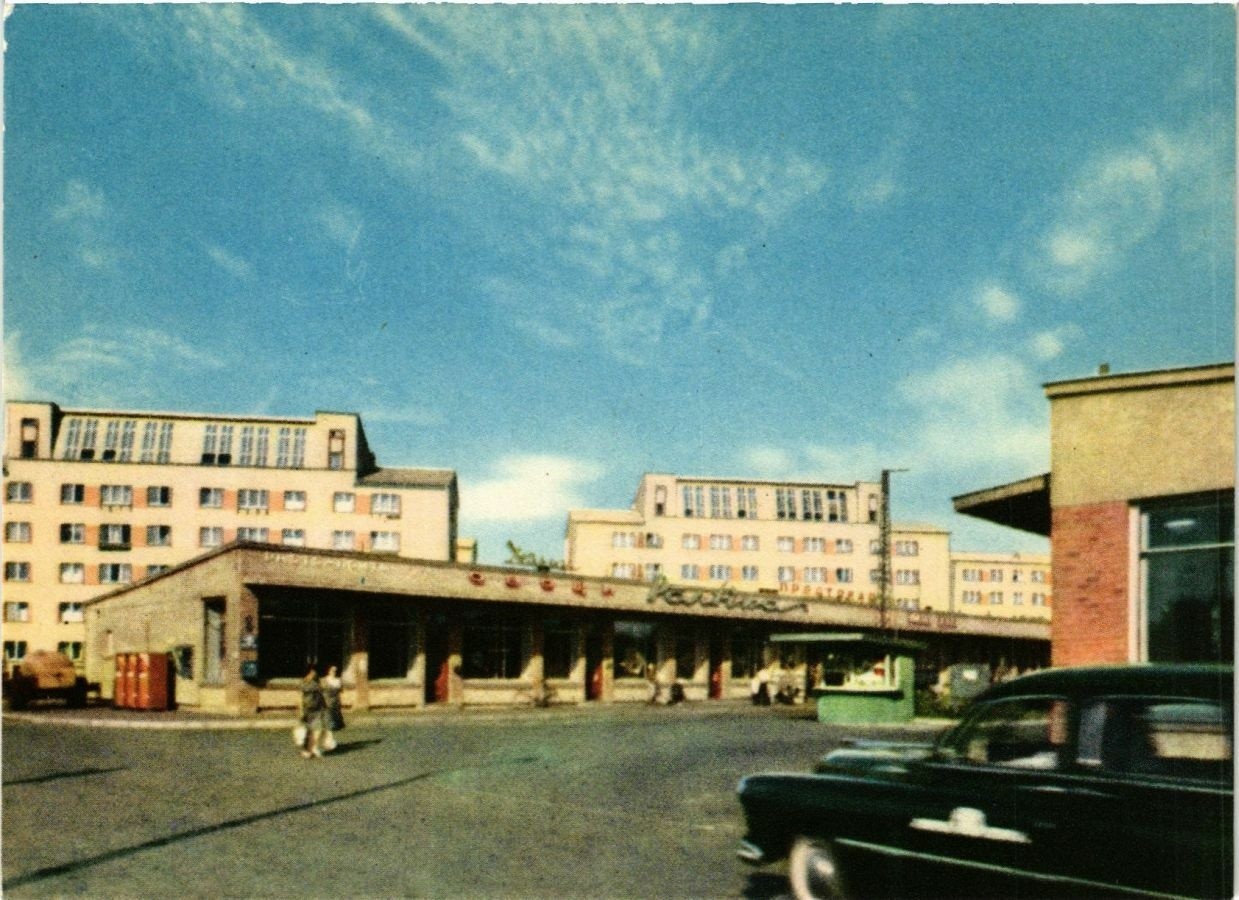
[8,653,87,711]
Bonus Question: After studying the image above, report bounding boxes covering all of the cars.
[737,661,1235,900]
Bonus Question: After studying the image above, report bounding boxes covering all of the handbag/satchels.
[293,722,308,746]
[321,729,340,751]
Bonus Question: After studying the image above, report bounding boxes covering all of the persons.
[297,662,327,760]
[751,661,824,708]
[967,700,1068,772]
[318,665,345,751]
